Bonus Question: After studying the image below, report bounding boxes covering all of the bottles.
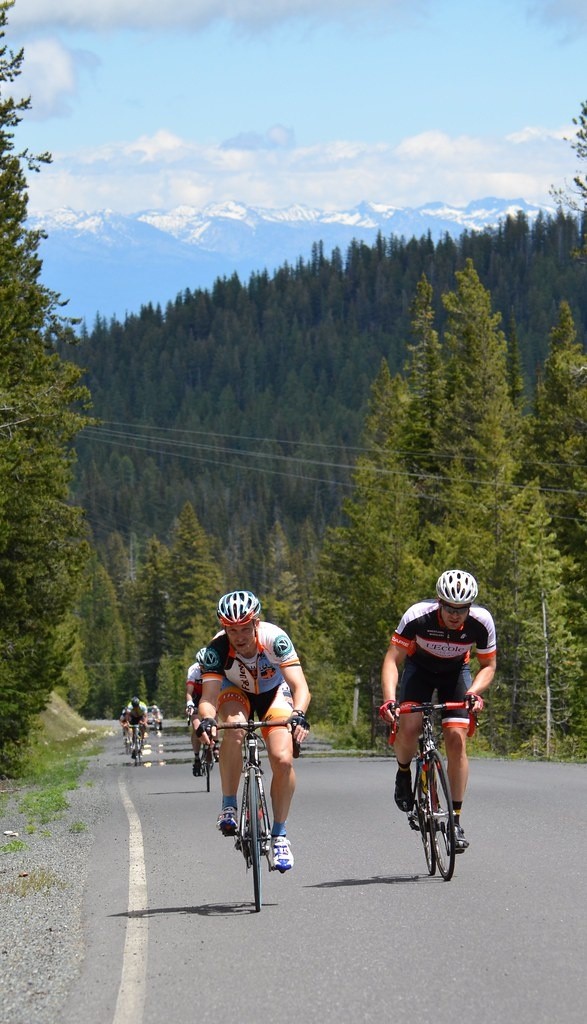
[246,793,263,826]
[418,762,423,783]
[422,759,437,795]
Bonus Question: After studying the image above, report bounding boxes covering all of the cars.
[147,705,164,724]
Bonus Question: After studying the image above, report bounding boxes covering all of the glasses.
[439,598,472,615]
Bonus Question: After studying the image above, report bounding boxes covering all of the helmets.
[195,647,207,666]
[131,697,139,705]
[435,570,478,604]
[216,590,262,627]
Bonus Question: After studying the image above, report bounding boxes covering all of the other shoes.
[213,749,220,762]
[192,759,201,776]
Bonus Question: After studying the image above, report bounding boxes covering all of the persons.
[119,696,161,750]
[378,570,497,852]
[186,648,219,777]
[199,591,311,870]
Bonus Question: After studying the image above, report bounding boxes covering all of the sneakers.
[394,768,414,812]
[215,806,239,830]
[270,836,295,870]
[446,821,470,850]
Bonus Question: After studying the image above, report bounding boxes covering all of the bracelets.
[186,700,194,706]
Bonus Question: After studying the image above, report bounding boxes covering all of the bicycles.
[206,720,300,912]
[188,704,219,792]
[122,724,146,766]
[389,694,480,881]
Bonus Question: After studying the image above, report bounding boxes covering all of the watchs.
[292,710,306,718]
